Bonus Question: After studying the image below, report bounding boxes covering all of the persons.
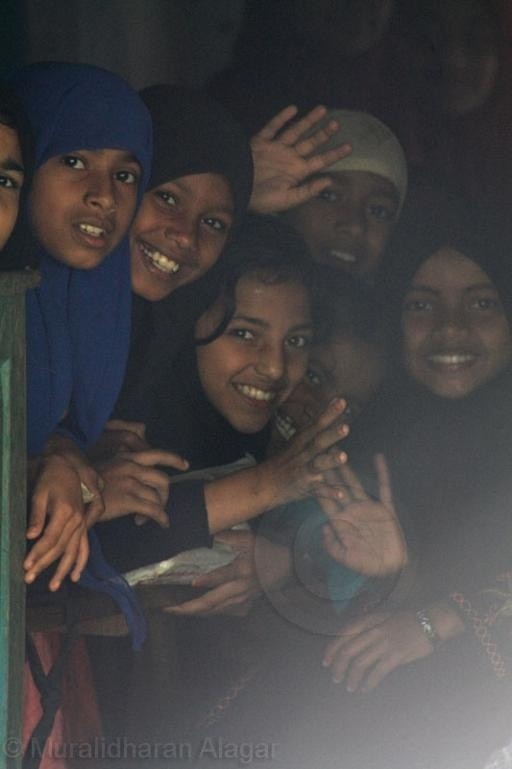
[0,62,512,769]
